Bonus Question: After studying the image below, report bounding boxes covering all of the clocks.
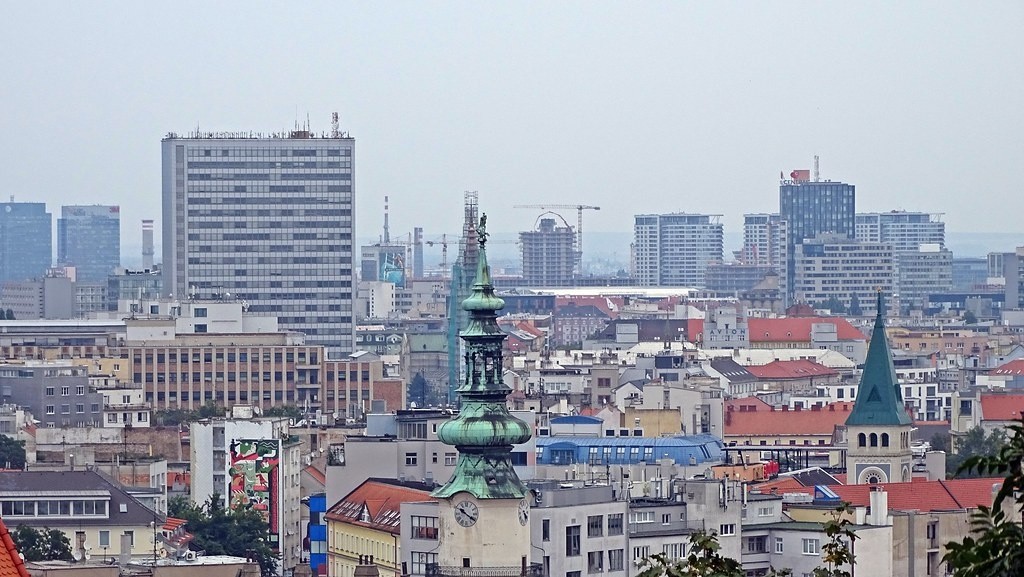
[518,499,530,527]
[454,501,479,527]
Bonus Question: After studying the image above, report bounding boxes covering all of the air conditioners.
[185,551,196,560]
